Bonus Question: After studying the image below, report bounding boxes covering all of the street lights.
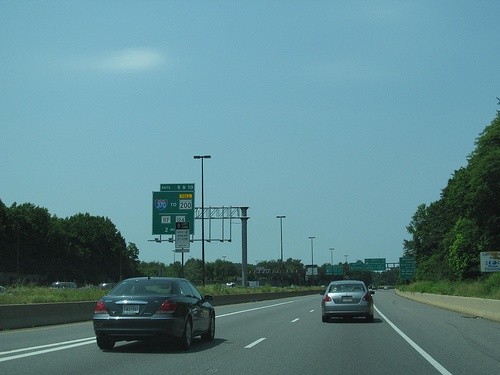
[309,236,315,276]
[192,154,212,287]
[344,254,349,262]
[276,215,286,262]
[329,248,334,275]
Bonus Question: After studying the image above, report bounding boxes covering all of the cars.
[51,282,77,289]
[320,280,376,323]
[92,276,217,352]
[97,282,117,289]
